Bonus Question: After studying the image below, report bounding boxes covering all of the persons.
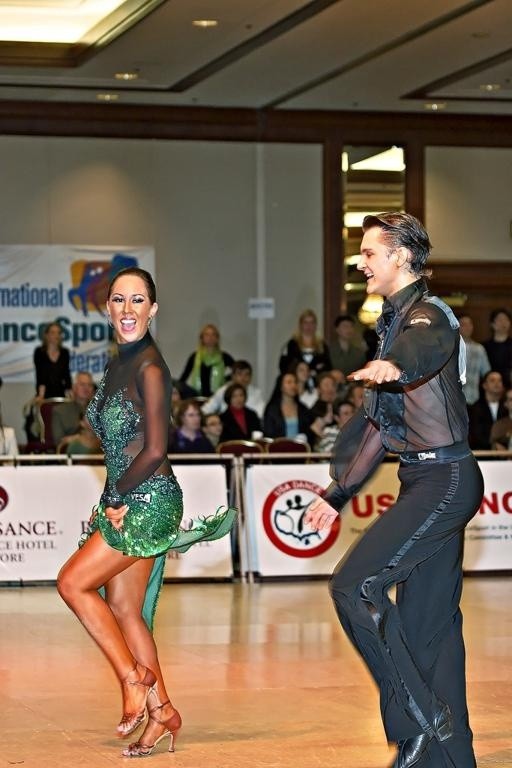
[221,386,262,441]
[179,325,235,398]
[52,371,94,447]
[491,390,512,453]
[480,309,511,391]
[59,407,103,454]
[190,415,223,454]
[306,213,485,768]
[200,362,268,418]
[168,402,217,454]
[329,316,368,381]
[310,373,337,417]
[278,311,332,373]
[297,363,320,406]
[58,270,184,762]
[169,379,180,435]
[348,385,368,409]
[468,372,503,449]
[34,323,74,407]
[263,373,315,444]
[450,314,493,423]
[315,400,357,454]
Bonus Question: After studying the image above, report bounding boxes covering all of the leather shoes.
[394,701,454,767]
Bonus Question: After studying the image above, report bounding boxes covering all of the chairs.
[57,435,80,454]
[37,397,84,453]
[265,438,312,463]
[216,438,264,466]
[170,397,209,432]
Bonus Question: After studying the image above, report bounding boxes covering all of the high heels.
[121,700,182,757]
[119,660,160,738]
